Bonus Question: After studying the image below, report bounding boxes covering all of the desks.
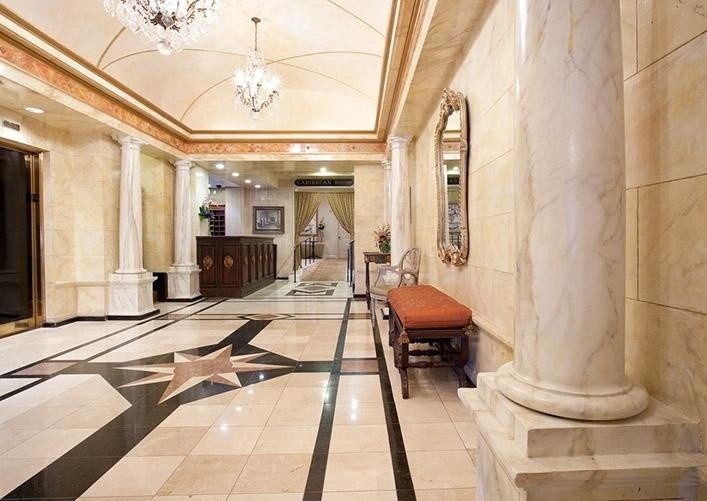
[360,251,391,311]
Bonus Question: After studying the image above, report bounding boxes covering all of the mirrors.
[430,84,470,269]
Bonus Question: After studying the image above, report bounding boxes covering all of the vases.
[378,241,389,254]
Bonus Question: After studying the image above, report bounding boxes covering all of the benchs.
[383,282,475,400]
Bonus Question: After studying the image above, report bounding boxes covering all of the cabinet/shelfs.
[207,204,225,237]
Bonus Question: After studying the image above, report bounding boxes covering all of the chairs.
[368,245,423,330]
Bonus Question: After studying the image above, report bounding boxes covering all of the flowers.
[198,205,216,222]
[371,224,392,252]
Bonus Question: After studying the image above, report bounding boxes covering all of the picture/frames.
[298,208,318,238]
[250,205,285,236]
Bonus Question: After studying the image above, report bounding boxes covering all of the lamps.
[228,15,284,120]
[101,0,224,59]
